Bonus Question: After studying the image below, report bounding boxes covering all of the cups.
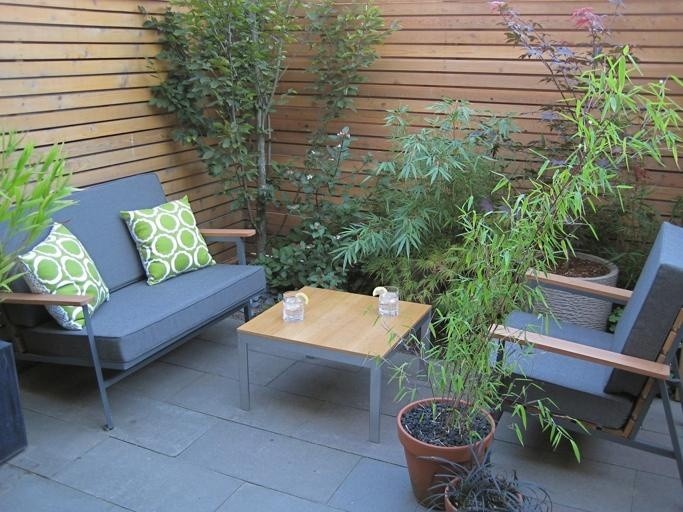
[282,290,304,322]
[377,286,399,317]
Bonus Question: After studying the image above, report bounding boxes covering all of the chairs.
[475,223,682,475]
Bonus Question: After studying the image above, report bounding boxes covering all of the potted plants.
[417,420,555,512]
[356,0,630,360]
[360,43,682,512]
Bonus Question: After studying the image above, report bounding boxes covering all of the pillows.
[17,193,219,337]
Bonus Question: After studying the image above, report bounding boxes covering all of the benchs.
[0,171,268,434]
